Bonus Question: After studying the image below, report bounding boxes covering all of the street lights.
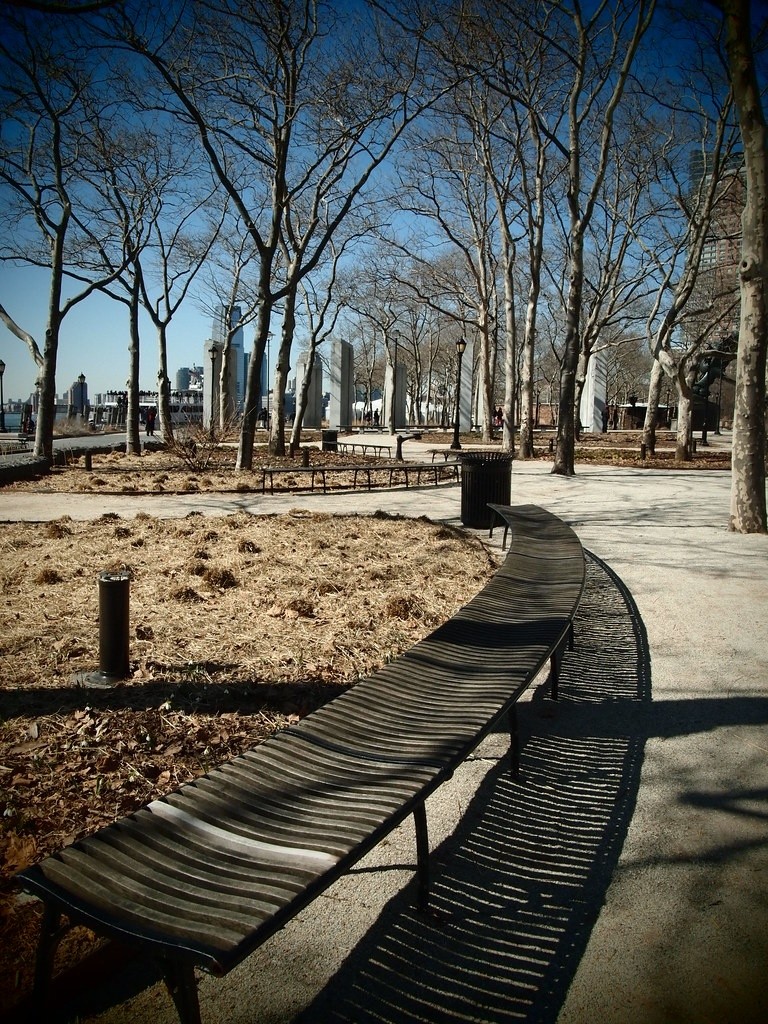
[714,326,729,436]
[265,331,276,432]
[208,343,219,443]
[450,335,467,451]
[78,372,86,419]
[391,330,401,435]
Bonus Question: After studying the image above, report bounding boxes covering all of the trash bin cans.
[457,451,516,529]
[321,429,339,451]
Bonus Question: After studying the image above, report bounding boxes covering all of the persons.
[613,410,619,430]
[257,407,268,429]
[25,416,34,434]
[365,411,372,425]
[492,406,503,425]
[373,411,380,426]
[601,405,610,433]
[289,411,295,427]
[140,406,157,436]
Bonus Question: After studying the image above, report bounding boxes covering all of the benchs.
[13,502,587,1024]
[536,424,590,433]
[336,442,392,458]
[336,425,388,435]
[300,425,326,432]
[260,448,461,498]
[403,424,451,435]
[472,424,521,434]
